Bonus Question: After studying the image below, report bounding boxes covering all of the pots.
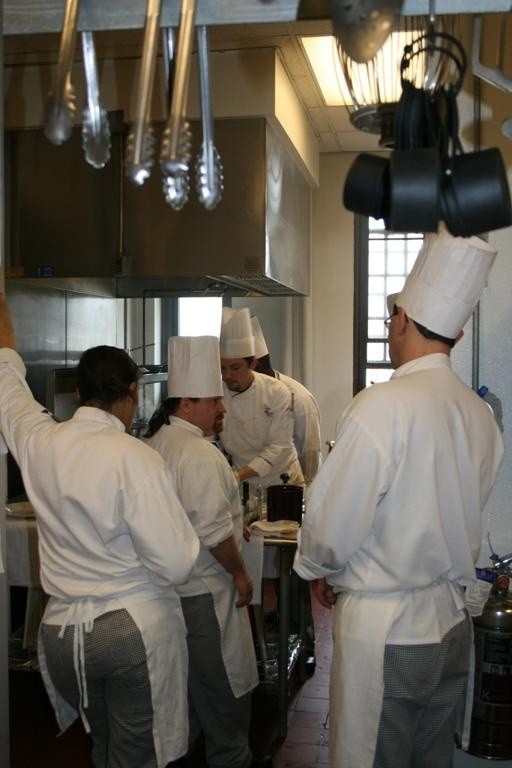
[342,79,512,239]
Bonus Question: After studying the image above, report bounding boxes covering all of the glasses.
[383,314,400,329]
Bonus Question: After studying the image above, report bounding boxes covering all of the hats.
[219,306,255,360]
[166,336,225,399]
[251,315,270,360]
[395,220,498,340]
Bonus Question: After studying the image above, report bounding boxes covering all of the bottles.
[241,481,264,520]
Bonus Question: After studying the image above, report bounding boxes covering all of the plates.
[138,365,164,373]
[6,501,35,518]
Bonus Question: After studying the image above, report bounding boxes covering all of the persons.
[139,397,261,768]
[250,354,320,490]
[1,292,203,767]
[204,358,305,503]
[293,302,498,768]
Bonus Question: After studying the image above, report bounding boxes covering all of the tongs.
[159,0,224,211]
[44,0,116,170]
[127,0,173,186]
